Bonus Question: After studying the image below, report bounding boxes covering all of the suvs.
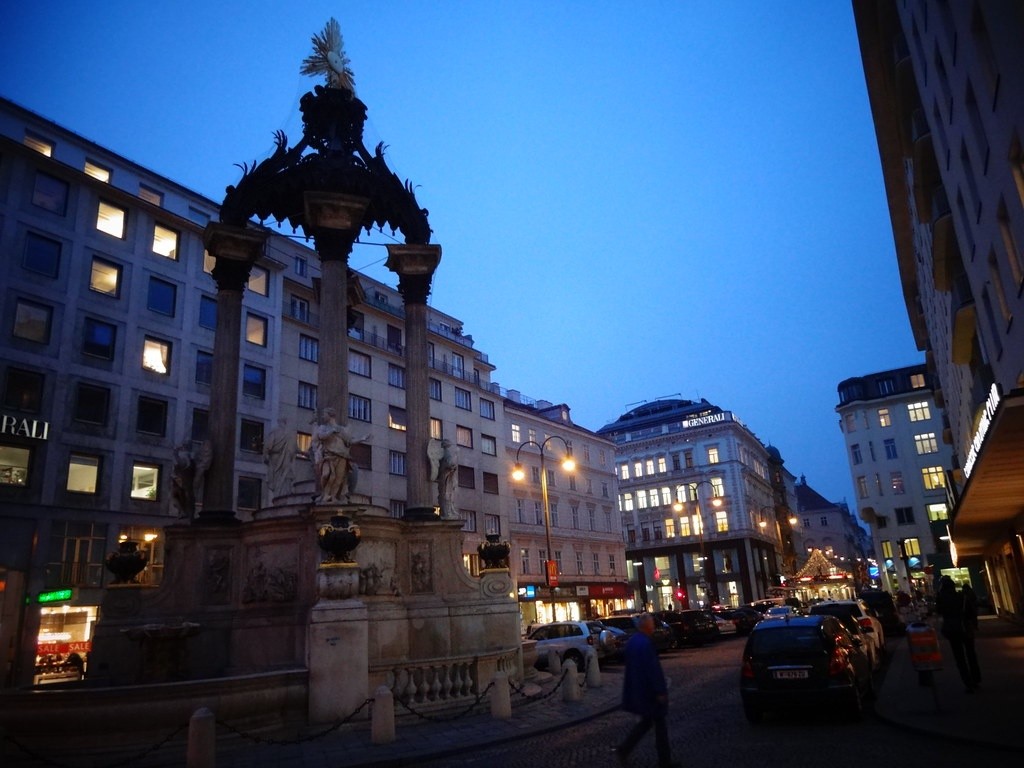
[528,621,617,671]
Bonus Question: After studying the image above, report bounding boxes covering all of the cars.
[598,598,803,664]
[741,615,865,719]
[808,598,885,662]
[840,617,880,672]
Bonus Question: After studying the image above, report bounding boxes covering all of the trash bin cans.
[906,623,941,670]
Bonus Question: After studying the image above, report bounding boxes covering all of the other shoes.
[964,687,976,694]
[613,747,627,768]
[667,760,683,768]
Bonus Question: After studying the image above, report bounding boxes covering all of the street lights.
[674,481,722,609]
[512,436,578,624]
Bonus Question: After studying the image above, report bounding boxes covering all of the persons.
[308,407,373,502]
[934,575,980,695]
[615,612,674,768]
[193,440,212,519]
[36,652,87,679]
[438,439,462,520]
[263,416,296,501]
